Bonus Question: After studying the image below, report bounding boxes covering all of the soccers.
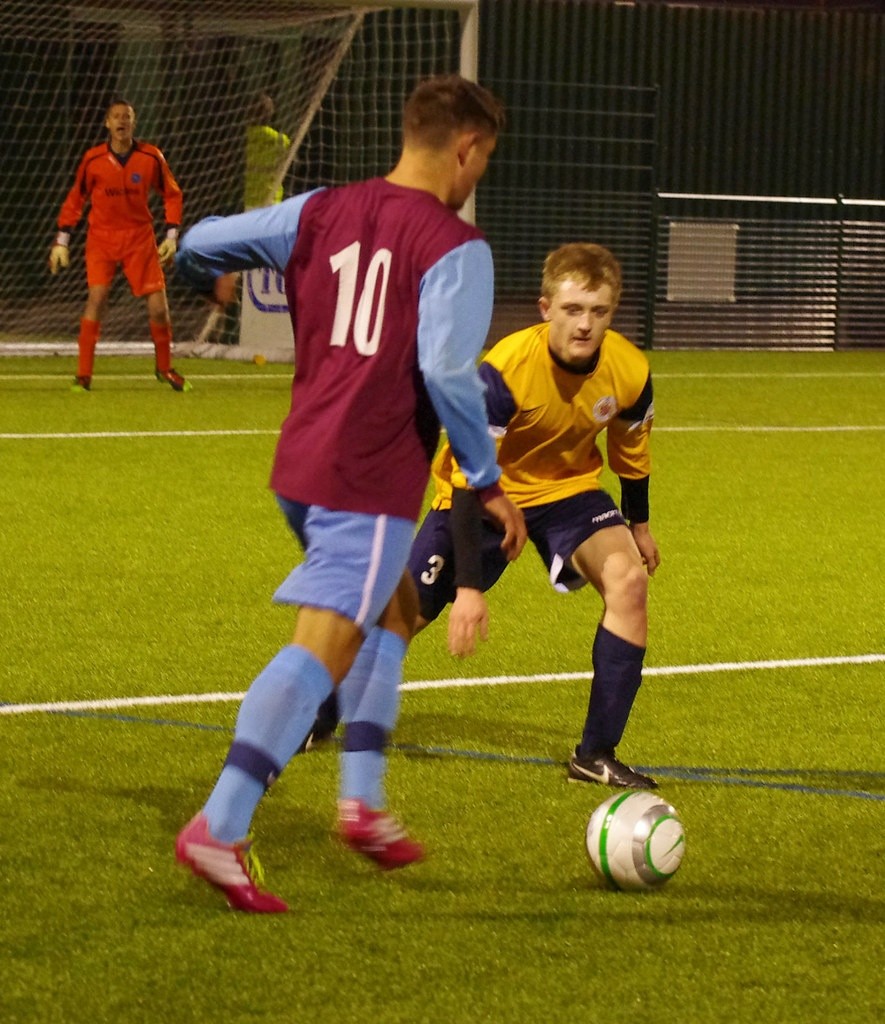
[584,790,686,893]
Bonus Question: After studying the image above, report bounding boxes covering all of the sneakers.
[297,716,340,754]
[155,368,193,392]
[70,375,91,393]
[339,799,424,871]
[568,747,658,790]
[176,811,289,914]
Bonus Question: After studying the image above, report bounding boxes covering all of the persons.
[236,88,298,213]
[50,99,197,393]
[176,73,525,913]
[303,242,665,788]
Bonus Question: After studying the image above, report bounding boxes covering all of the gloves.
[157,239,176,264]
[50,245,70,275]
